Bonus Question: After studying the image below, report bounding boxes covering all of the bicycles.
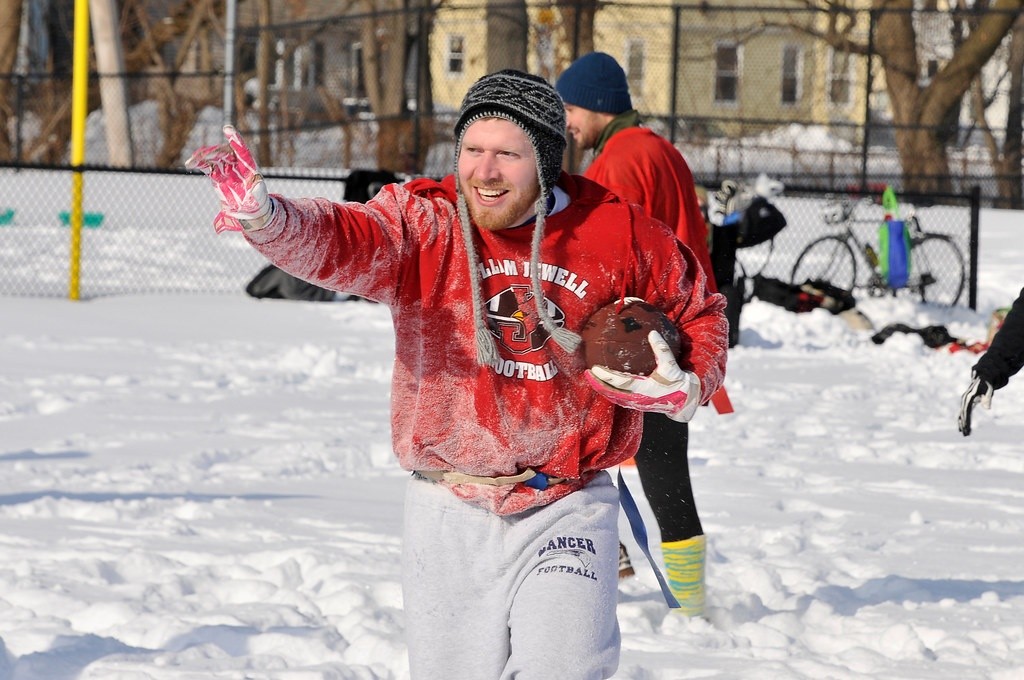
[790,191,965,308]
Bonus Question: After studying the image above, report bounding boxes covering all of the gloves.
[958,370,994,437]
[584,330,701,423]
[185,125,278,235]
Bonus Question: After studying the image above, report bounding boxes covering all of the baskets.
[818,200,850,225]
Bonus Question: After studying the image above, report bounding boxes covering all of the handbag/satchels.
[878,220,912,289]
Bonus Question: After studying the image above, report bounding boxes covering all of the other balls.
[579,296,682,391]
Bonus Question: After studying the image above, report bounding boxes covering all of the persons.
[555,51,719,617]
[694,180,787,348]
[957,288,1024,436]
[183,68,728,680]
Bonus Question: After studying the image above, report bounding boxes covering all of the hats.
[453,68,582,367]
[555,52,632,114]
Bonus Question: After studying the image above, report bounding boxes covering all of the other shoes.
[618,544,635,578]
